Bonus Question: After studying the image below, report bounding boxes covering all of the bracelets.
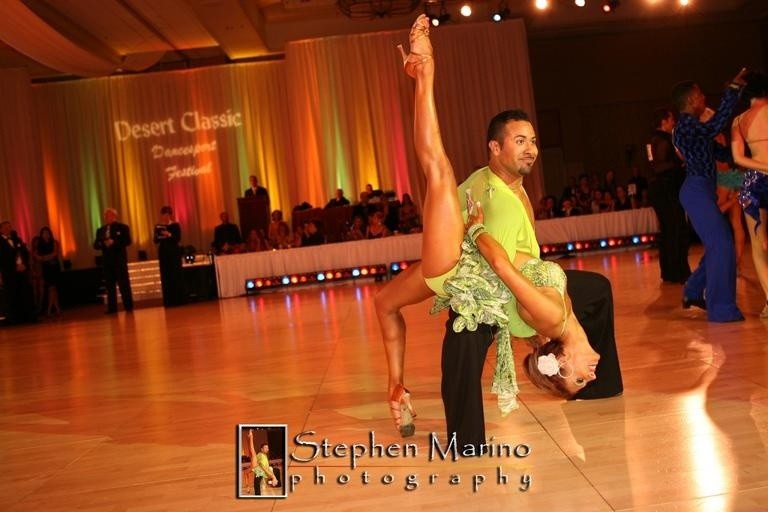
[467,223,487,245]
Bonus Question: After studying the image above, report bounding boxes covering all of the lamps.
[432,0,451,27]
[492,0,511,22]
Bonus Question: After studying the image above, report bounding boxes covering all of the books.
[155,223,167,236]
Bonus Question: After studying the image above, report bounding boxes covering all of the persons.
[242,427,278,493]
[533,160,649,221]
[339,213,363,242]
[91,206,133,315]
[253,443,281,496]
[672,65,748,323]
[211,211,241,255]
[301,218,325,246]
[440,107,624,457]
[376,194,398,232]
[324,187,350,208]
[351,191,378,234]
[243,175,269,207]
[373,11,600,438]
[28,235,44,305]
[394,191,419,233]
[363,214,388,283]
[361,183,383,198]
[697,106,747,279]
[641,105,691,286]
[266,209,290,248]
[153,205,188,309]
[32,225,61,318]
[0,220,42,326]
[232,224,273,254]
[729,72,766,320]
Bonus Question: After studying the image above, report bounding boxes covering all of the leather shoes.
[760,301,768,317]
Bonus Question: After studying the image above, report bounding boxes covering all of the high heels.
[681,295,706,310]
[397,14,434,78]
[388,383,416,438]
